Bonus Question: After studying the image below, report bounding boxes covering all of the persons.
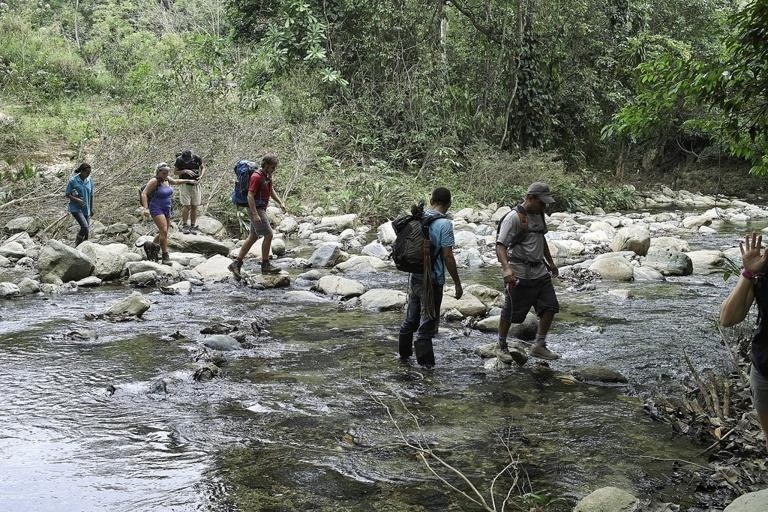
[173,149,206,235]
[717,233,768,460]
[494,182,564,363]
[137,162,199,266]
[64,163,95,248]
[229,152,288,281]
[398,187,464,369]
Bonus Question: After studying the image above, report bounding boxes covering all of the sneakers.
[494,343,514,364]
[530,342,557,359]
[190,226,197,234]
[181,224,190,234]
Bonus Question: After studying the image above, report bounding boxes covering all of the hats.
[526,182,556,203]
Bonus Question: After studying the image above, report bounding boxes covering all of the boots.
[162,252,173,266]
[227,260,241,280]
[261,259,282,273]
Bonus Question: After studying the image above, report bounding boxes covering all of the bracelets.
[143,207,149,210]
[741,269,757,281]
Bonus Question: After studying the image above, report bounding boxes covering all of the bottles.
[71,187,79,198]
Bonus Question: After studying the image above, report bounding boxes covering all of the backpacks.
[391,198,446,272]
[232,159,272,207]
[496,204,528,263]
[138,175,160,208]
